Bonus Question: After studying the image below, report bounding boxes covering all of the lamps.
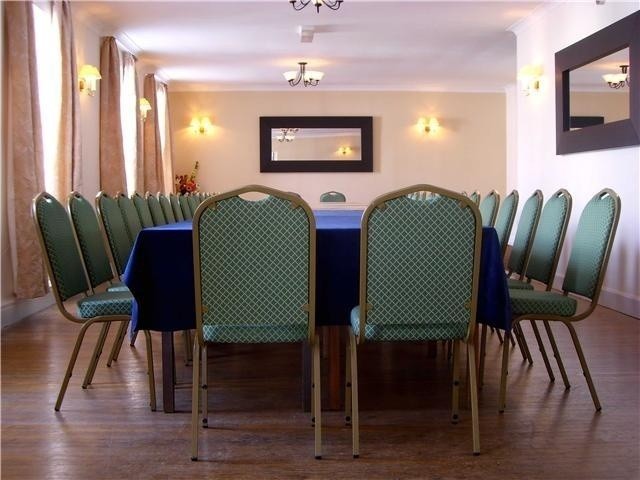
[283,61,324,89]
[79,62,103,98]
[276,128,299,143]
[187,116,214,137]
[134,96,153,122]
[601,64,629,90]
[518,63,545,98]
[414,115,441,136]
[335,145,352,155]
[290,1,345,15]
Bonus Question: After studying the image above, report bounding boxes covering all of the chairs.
[33,183,222,416]
[188,181,324,461]
[346,183,484,461]
[403,182,621,418]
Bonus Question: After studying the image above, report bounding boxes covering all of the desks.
[122,208,517,415]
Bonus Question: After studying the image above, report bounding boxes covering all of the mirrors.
[260,117,373,174]
[555,12,640,155]
